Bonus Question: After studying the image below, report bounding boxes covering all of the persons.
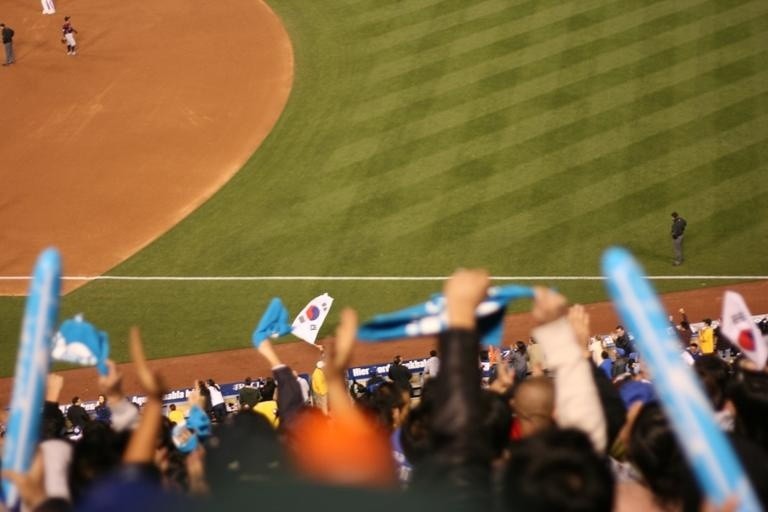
[0,267,768,510]
[0,22,17,67]
[668,210,687,267]
[39,0,57,17]
[60,15,80,56]
